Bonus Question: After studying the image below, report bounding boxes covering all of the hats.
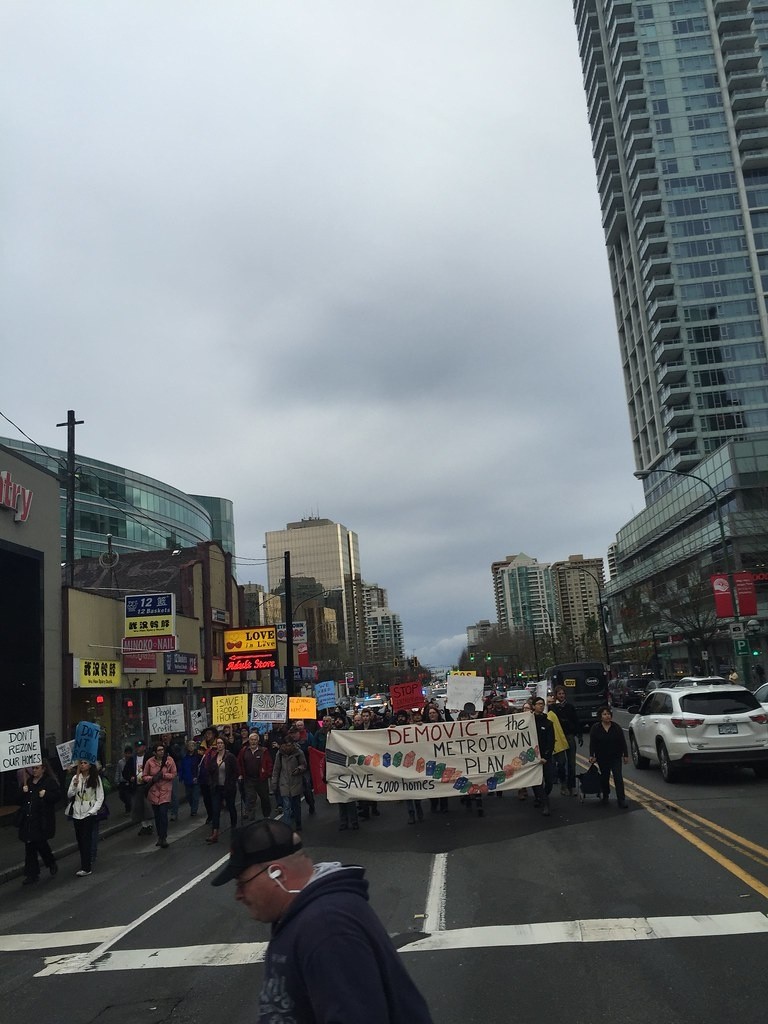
[397,710,407,717]
[136,740,145,745]
[210,818,304,887]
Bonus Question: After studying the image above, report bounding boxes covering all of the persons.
[729,669,738,685]
[210,818,432,1024]
[589,708,628,809]
[15,684,584,887]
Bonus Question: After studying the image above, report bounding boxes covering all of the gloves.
[578,738,583,747]
[153,773,162,782]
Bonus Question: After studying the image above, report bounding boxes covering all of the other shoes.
[431,809,439,813]
[338,825,348,832]
[440,809,449,813]
[466,801,472,809]
[560,788,577,796]
[418,817,424,824]
[50,864,57,875]
[478,808,485,817]
[22,875,39,885]
[353,824,359,829]
[533,797,540,807]
[309,804,315,814]
[138,825,153,835]
[156,838,169,849]
[76,870,92,876]
[408,815,415,824]
[206,829,218,843]
[618,799,628,808]
[542,800,549,815]
[518,790,528,800]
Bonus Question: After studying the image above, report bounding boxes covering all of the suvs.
[629,684,768,782]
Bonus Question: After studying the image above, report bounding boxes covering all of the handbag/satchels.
[577,764,609,793]
[144,782,151,799]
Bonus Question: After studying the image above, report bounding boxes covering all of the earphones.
[269,870,282,880]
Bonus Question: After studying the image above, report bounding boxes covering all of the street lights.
[287,586,345,704]
[522,602,557,673]
[557,565,612,678]
[635,469,754,690]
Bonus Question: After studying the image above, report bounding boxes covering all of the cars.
[353,692,394,716]
[503,691,532,710]
[609,673,768,725]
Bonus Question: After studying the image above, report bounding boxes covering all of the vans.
[544,663,612,731]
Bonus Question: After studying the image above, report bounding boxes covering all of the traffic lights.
[487,653,491,661]
[519,671,522,677]
[470,653,475,663]
